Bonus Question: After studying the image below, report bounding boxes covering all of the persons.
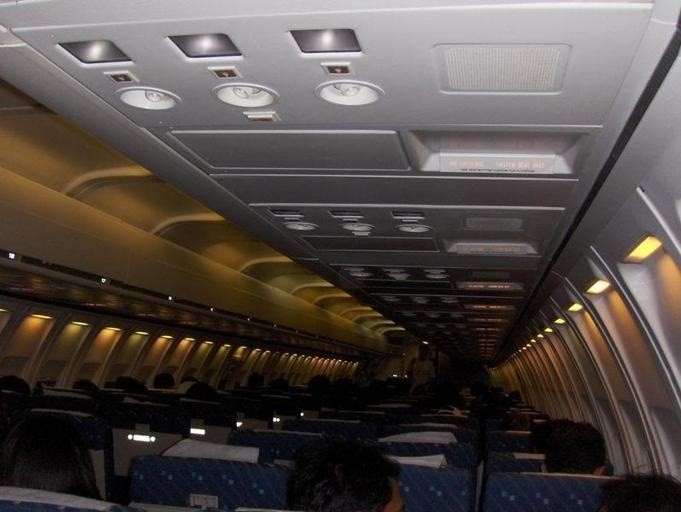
[1,410,107,501]
[1,365,617,477]
[285,459,407,512]
[406,344,438,395]
[593,473,681,510]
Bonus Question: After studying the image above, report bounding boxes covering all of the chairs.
[0,363,680,512]
